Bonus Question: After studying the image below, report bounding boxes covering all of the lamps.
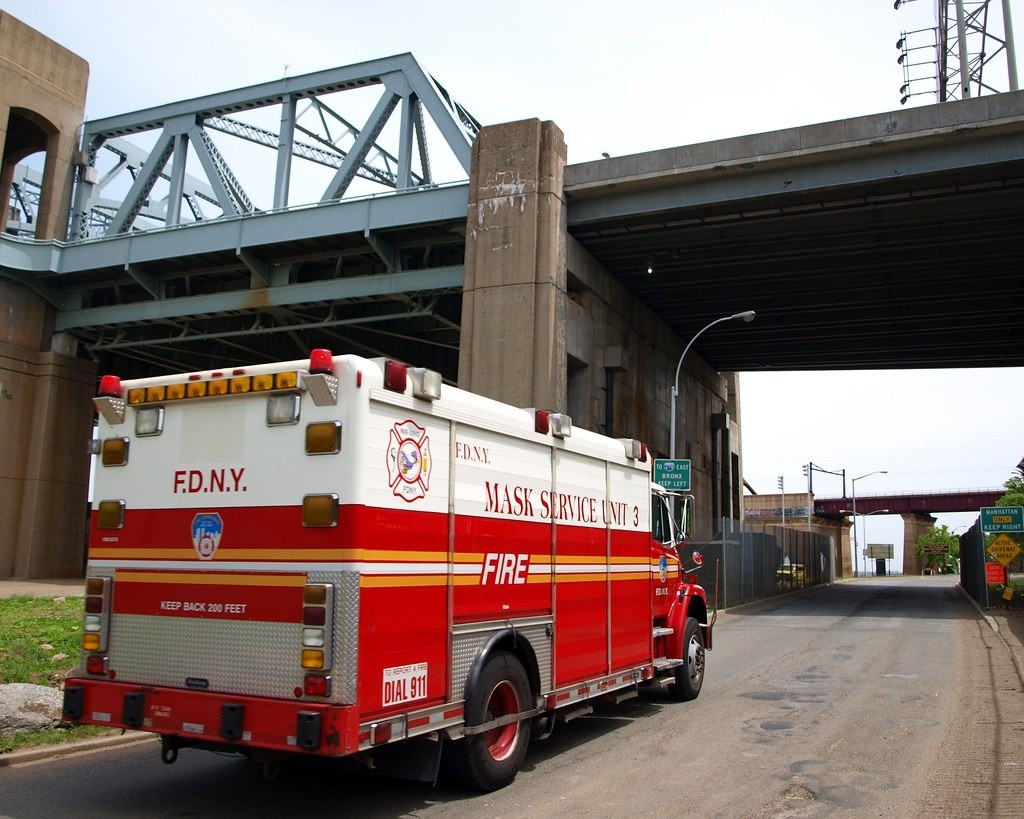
[894,0,939,106]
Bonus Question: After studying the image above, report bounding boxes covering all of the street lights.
[838,508,892,578]
[852,470,889,577]
[670,309,759,532]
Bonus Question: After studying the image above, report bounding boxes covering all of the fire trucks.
[56,340,714,798]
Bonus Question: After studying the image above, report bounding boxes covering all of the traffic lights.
[801,463,810,477]
[778,477,784,491]
